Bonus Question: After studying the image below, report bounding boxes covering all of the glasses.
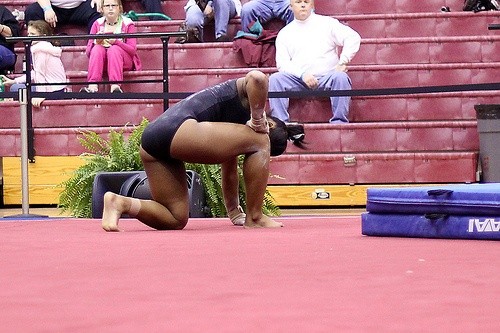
[102,4,118,9]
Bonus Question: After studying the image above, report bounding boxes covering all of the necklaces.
[106,21,118,25]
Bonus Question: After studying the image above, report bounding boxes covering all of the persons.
[79,0,141,94]
[24,0,103,34]
[0,4,21,75]
[0,21,67,108]
[183,0,242,41]
[268,0,361,123]
[140,0,163,21]
[241,0,294,32]
[102,69,310,231]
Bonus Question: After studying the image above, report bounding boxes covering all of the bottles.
[0,76,5,101]
[22,56,27,73]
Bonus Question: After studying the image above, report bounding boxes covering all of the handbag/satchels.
[175,22,202,44]
[55,32,75,46]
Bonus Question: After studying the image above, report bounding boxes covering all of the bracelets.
[44,5,52,11]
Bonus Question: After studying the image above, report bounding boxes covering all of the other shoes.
[110,84,124,93]
[216,35,230,42]
[78,84,98,94]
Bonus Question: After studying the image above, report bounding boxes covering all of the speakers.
[92,169,208,219]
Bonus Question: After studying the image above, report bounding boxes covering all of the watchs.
[1,24,4,34]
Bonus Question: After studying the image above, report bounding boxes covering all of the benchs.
[0,0,500,184]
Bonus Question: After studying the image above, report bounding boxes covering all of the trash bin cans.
[473,103,500,183]
